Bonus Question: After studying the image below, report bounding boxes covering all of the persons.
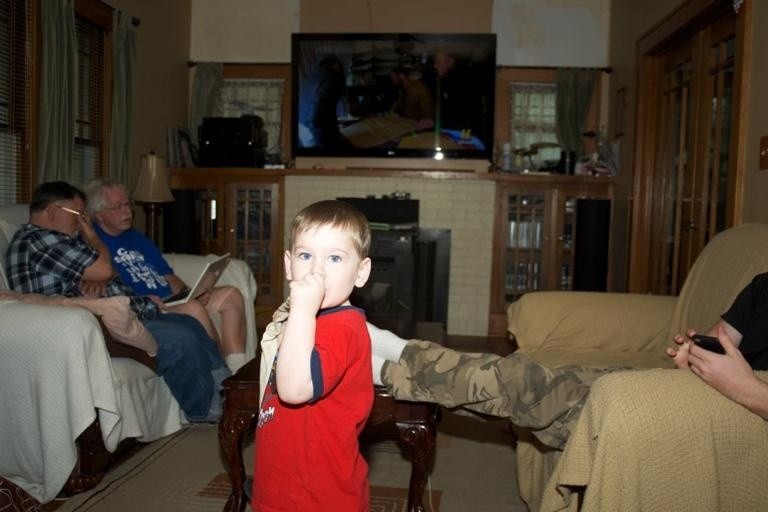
[390,46,475,131]
[250,200,375,512]
[366,272,768,451]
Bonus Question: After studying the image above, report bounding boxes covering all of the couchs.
[0,205,259,494]
[504,223,768,509]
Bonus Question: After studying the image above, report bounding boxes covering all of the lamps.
[131,151,177,246]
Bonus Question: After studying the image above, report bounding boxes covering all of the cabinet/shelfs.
[169,169,286,329]
[489,180,613,339]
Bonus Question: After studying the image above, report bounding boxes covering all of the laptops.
[163,252,231,307]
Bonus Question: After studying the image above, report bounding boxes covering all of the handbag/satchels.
[197,117,268,169]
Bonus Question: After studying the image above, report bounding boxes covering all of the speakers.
[159,187,205,254]
[569,198,615,293]
[338,197,419,231]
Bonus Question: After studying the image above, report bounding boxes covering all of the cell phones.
[692,335,726,354]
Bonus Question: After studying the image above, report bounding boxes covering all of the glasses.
[56,206,85,220]
[104,202,134,212]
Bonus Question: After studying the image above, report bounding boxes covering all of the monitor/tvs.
[291,33,496,158]
